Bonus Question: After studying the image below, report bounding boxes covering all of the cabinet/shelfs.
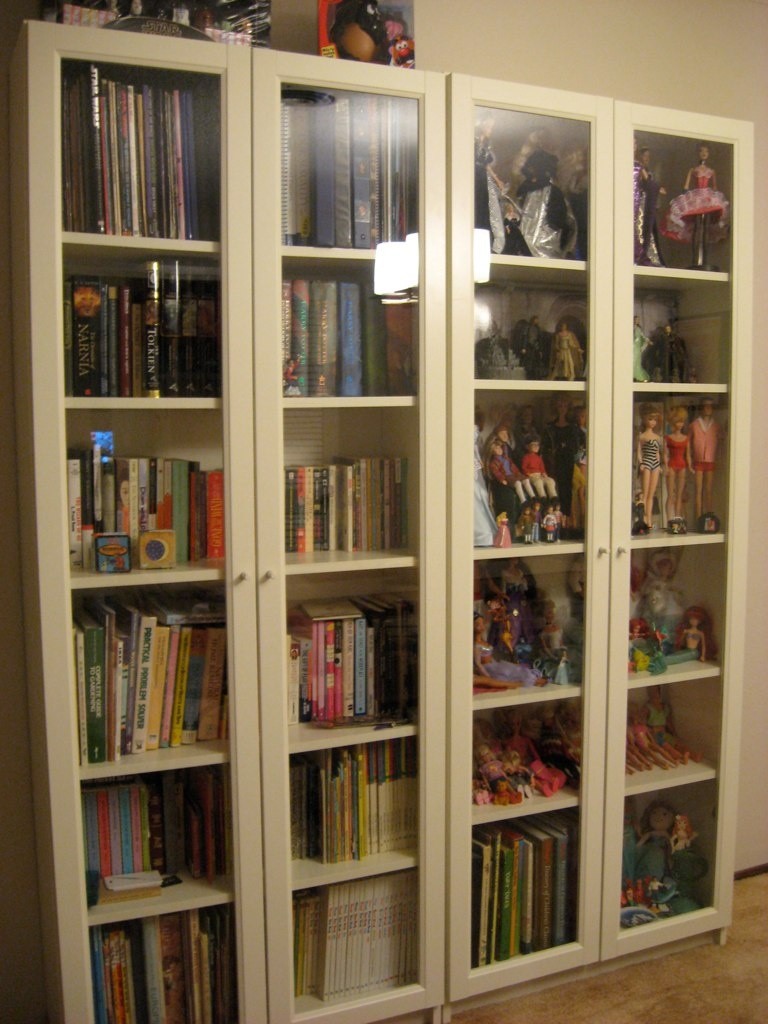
[0,23,753,1024]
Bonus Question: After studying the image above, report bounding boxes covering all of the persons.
[619,145,719,931]
[470,98,589,806]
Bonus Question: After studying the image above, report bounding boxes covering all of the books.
[477,818,582,974]
[58,53,420,1024]
